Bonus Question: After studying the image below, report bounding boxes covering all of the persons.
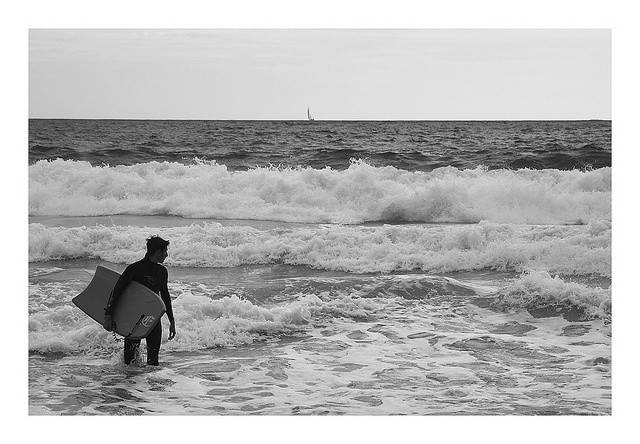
[104,234,175,364]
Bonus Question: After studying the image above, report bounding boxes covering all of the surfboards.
[72,265,166,340]
